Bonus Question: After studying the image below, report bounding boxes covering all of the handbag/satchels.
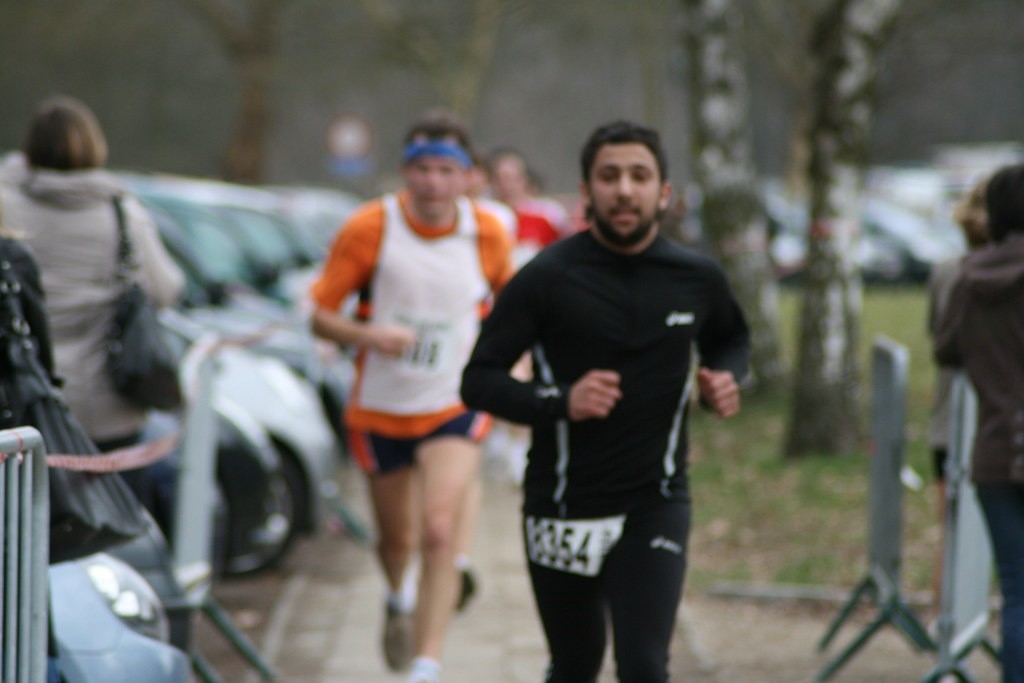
[107,197,184,409]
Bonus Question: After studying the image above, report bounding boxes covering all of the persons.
[928,162,1024,683]
[459,120,751,683]
[310,119,571,683]
[0,96,192,656]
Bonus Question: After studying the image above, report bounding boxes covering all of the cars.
[755,139,1023,287]
[2,171,369,683]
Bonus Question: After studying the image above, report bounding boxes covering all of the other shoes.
[384,601,415,672]
[457,566,476,610]
